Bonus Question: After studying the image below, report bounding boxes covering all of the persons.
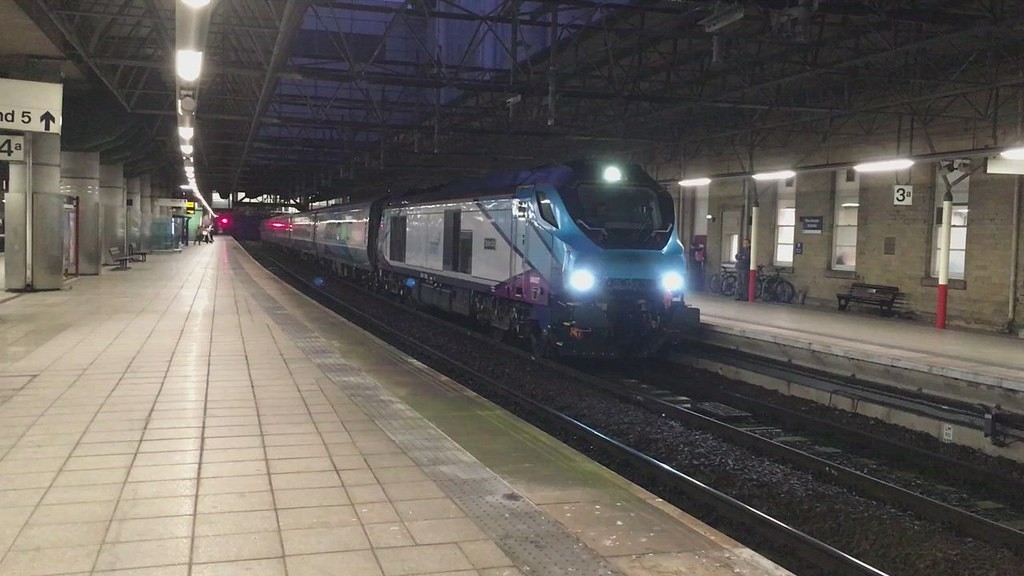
[688,242,703,291]
[194,224,214,245]
[731,238,751,301]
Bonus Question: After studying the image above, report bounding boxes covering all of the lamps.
[180,89,196,118]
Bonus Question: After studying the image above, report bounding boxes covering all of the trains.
[259,163,689,368]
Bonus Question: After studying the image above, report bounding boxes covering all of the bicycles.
[708,263,796,303]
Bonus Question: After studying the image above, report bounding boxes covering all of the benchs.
[108,246,132,271]
[128,241,147,263]
[837,281,900,317]
